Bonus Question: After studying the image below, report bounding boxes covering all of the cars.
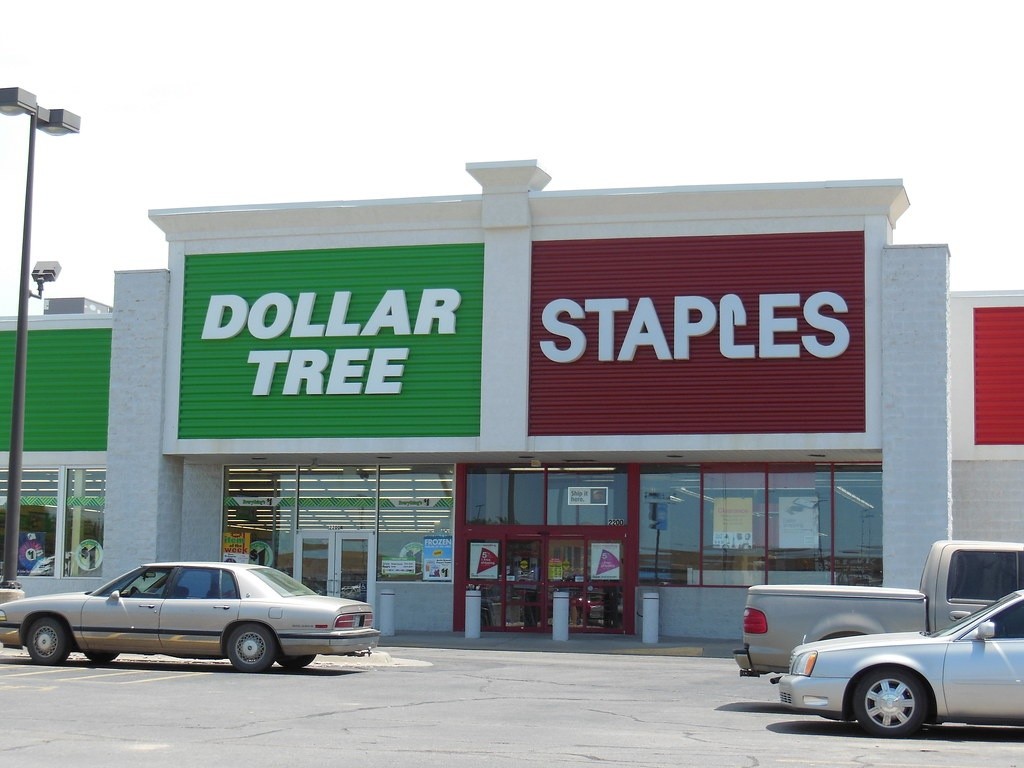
[777,589,1023,735]
[0,562,381,675]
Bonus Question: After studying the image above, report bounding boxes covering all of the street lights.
[0,86,83,599]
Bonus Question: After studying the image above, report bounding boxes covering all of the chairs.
[173,586,189,598]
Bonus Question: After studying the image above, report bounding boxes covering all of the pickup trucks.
[731,539,1024,683]
